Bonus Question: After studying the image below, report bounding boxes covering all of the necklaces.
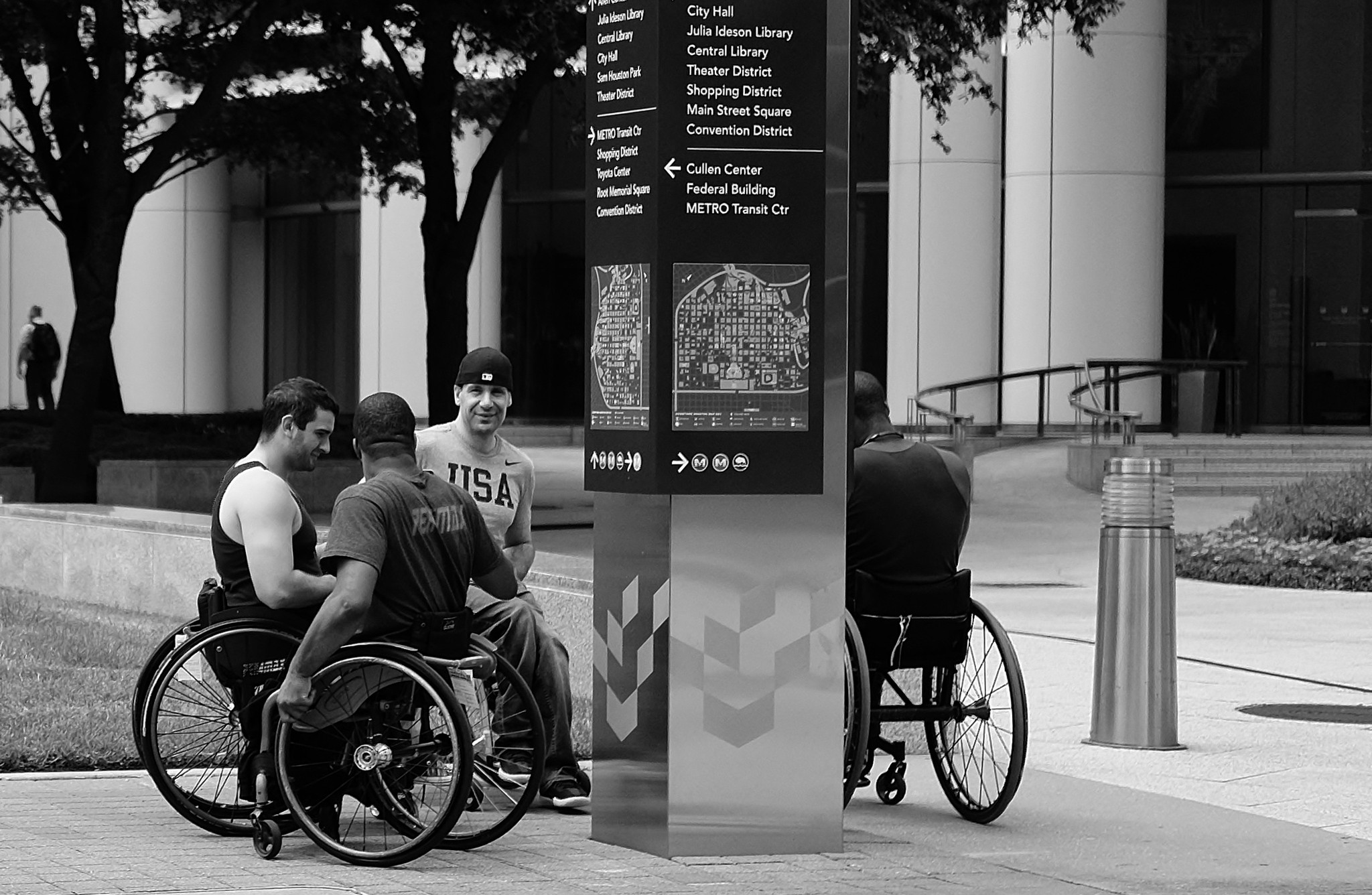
[862,431,903,446]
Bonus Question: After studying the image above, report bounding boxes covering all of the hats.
[456,348,515,388]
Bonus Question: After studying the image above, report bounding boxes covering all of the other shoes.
[493,755,535,782]
[540,778,592,808]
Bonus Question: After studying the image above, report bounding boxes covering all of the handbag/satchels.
[29,321,60,362]
[407,607,479,658]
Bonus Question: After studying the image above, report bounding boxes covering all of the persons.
[413,346,592,806]
[847,370,973,749]
[210,378,520,723]
[15,305,59,412]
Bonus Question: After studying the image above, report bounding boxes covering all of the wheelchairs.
[842,561,1030,825]
[130,575,550,868]
